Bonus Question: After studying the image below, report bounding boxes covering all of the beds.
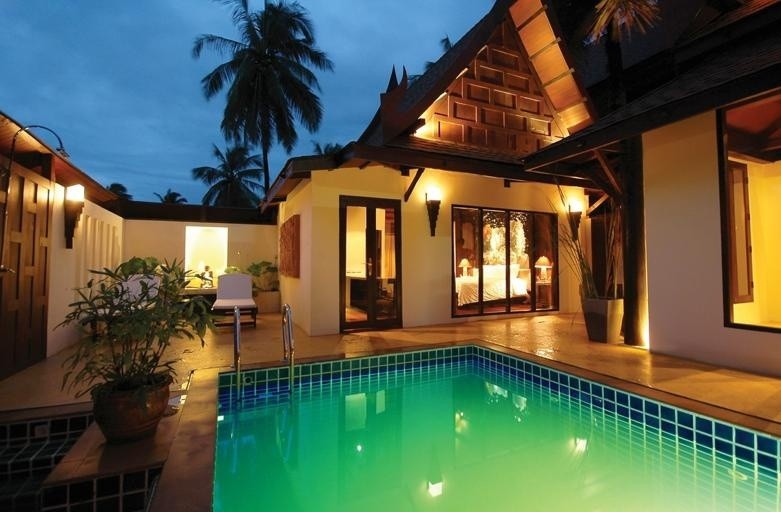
[456,268,532,310]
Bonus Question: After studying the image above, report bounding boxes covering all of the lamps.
[567,208,584,242]
[459,259,473,278]
[64,182,85,250]
[426,198,442,235]
[536,258,550,279]
[2,125,70,272]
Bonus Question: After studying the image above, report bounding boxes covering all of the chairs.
[82,270,160,349]
[213,272,260,330]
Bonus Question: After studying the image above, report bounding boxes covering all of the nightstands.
[535,283,551,306]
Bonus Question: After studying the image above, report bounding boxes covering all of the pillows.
[484,264,520,279]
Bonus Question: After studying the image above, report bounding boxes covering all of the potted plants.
[541,172,625,343]
[59,254,214,441]
[250,260,283,314]
